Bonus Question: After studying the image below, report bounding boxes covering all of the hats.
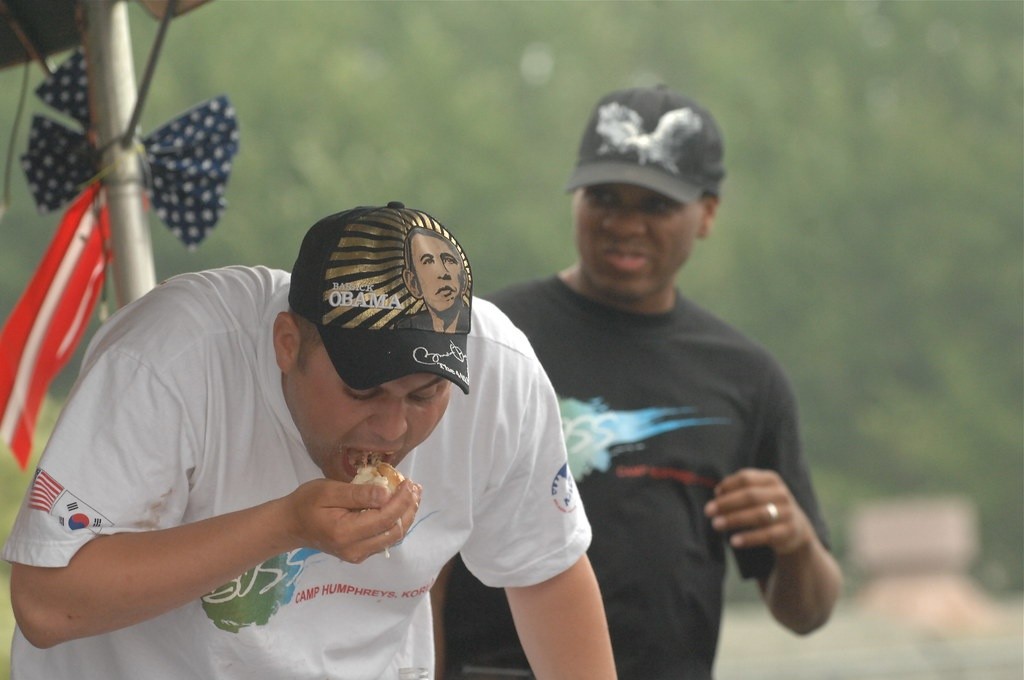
[565,85,724,206]
[289,203,472,393]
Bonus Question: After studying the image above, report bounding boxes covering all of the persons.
[3,202,619,680]
[429,83,841,680]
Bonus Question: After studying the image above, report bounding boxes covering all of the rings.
[766,502,779,523]
